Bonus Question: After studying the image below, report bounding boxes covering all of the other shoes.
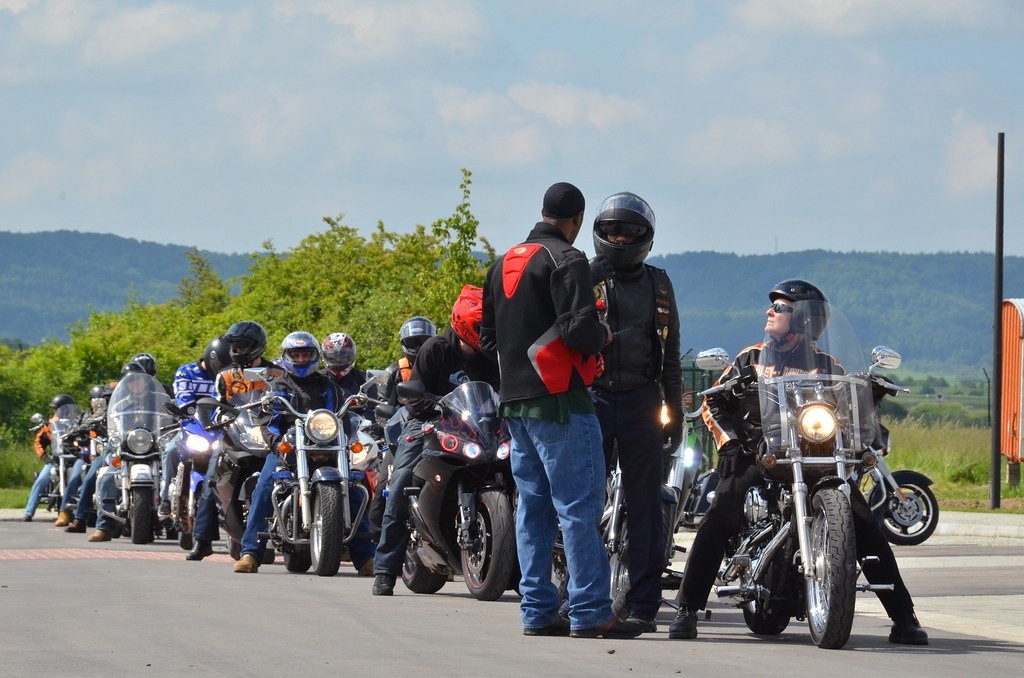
[358,559,374,576]
[625,611,657,632]
[186,539,213,560]
[22,509,111,542]
[233,554,258,572]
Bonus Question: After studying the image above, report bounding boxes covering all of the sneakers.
[372,573,396,595]
[523,615,571,636]
[569,615,644,639]
[506,573,525,597]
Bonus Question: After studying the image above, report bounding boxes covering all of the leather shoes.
[669,606,699,639]
[889,616,928,645]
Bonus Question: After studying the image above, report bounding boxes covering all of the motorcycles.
[27,364,939,604]
[693,298,912,650]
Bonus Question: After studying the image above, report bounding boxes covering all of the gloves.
[717,441,744,479]
[869,372,897,406]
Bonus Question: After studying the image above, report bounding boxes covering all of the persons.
[559,188,683,631]
[476,184,644,639]
[358,314,437,575]
[185,322,286,562]
[319,332,377,419]
[370,284,500,593]
[668,278,930,644]
[156,337,234,516]
[233,329,339,572]
[21,351,174,541]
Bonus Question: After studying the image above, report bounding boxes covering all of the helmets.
[593,192,656,269]
[450,284,483,351]
[768,279,830,338]
[48,320,357,409]
[399,316,437,360]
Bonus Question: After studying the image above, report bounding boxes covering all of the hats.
[542,182,586,219]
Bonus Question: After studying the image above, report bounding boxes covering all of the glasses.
[771,303,793,313]
